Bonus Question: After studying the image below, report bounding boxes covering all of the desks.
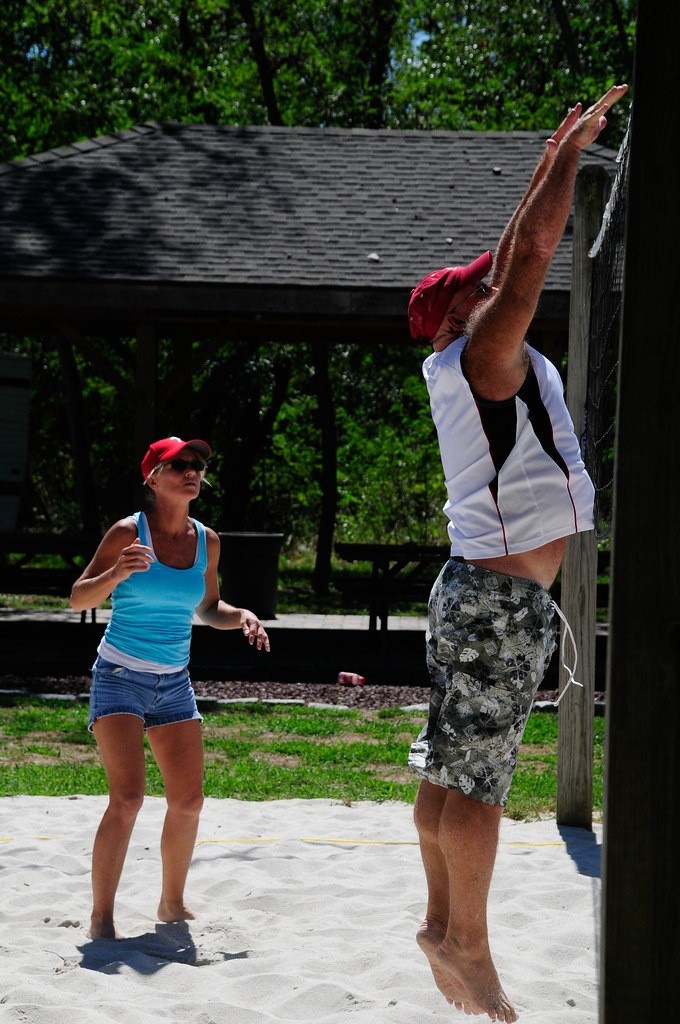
[334,540,451,629]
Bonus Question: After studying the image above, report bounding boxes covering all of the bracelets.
[547,138,557,147]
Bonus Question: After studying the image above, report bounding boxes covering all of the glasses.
[143,458,206,485]
[447,283,491,314]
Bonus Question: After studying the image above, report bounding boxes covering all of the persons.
[406,82,628,1024]
[70,437,270,940]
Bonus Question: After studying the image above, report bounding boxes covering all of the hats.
[408,250,493,342]
[141,437,211,475]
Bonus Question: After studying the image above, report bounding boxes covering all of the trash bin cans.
[217,531,284,620]
